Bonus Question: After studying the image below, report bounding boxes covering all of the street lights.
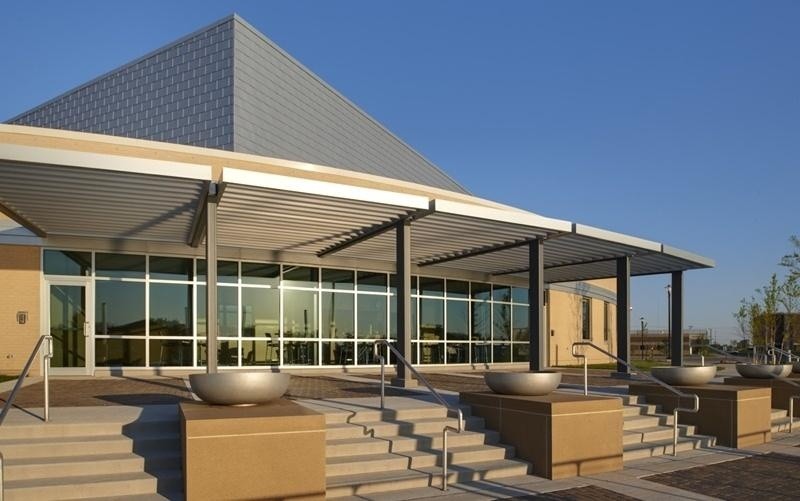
[639,317,644,360]
[664,284,672,360]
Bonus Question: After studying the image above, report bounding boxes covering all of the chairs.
[266,330,313,365]
